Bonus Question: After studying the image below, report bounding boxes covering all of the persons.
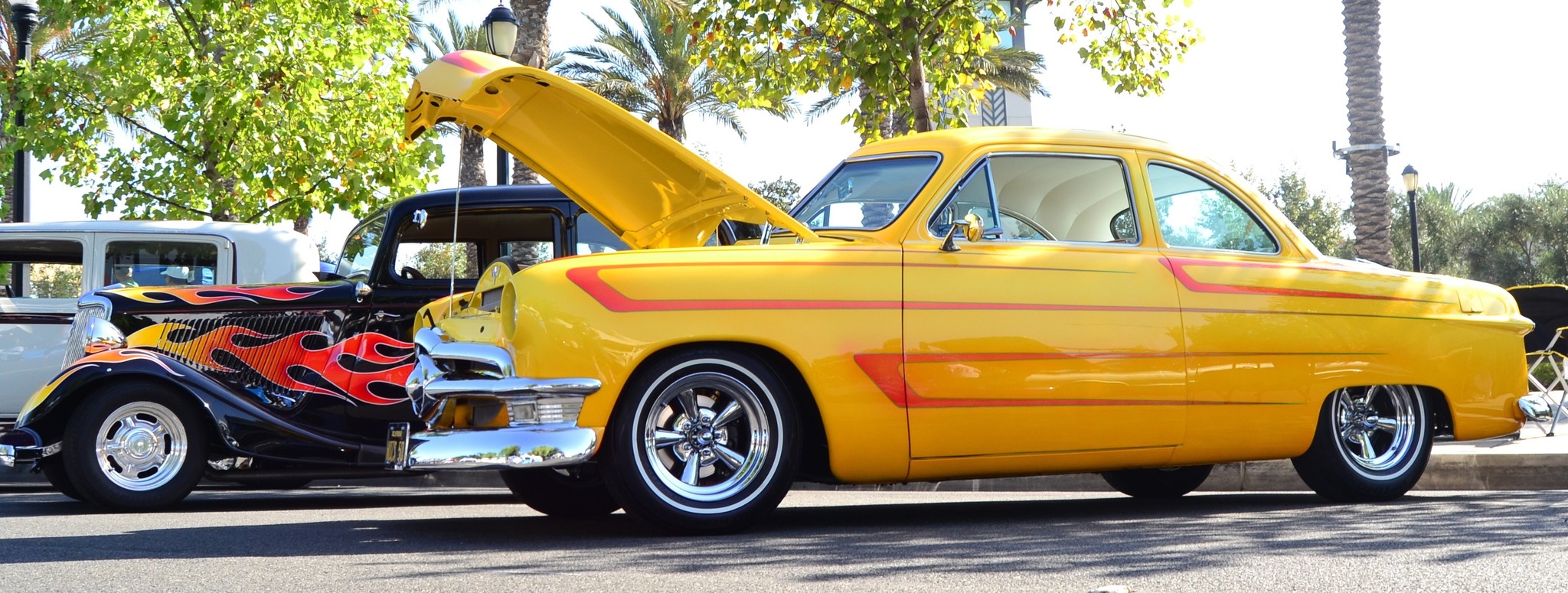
[160,266,189,285]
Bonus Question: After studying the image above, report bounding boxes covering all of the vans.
[0,218,321,437]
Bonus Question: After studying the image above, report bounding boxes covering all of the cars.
[383,49,1553,548]
[1,183,766,516]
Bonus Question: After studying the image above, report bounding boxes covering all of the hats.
[160,266,189,279]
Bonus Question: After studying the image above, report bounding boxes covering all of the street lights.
[7,0,45,298]
[480,1,519,186]
[1401,164,1422,273]
